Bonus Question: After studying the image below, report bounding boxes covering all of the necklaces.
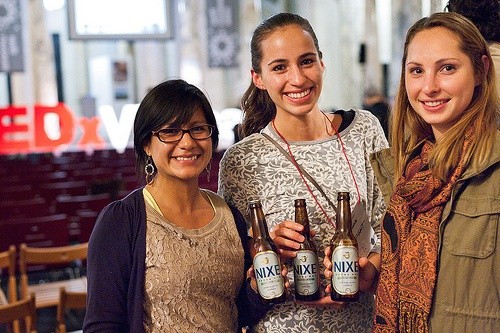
[271,110,361,227]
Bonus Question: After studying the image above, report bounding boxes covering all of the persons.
[82,79,291,333]
[215,14,389,333]
[325,12,500,333]
[443,1,500,73]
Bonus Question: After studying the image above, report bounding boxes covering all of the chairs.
[0,147,230,333]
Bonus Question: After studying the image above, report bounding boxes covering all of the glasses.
[152,124,216,143]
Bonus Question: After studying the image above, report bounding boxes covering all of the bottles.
[292,199,320,302]
[248,200,287,305]
[330,191,360,303]
